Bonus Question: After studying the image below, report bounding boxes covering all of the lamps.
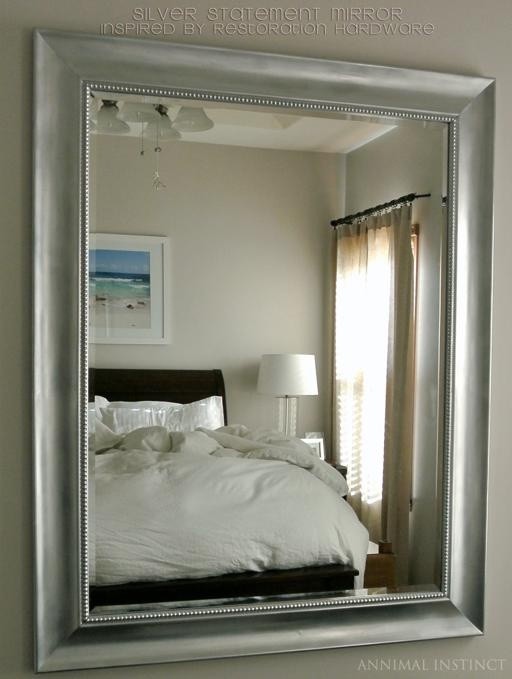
[257,353,319,438]
[88,86,214,190]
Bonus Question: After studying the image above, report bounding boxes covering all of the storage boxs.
[364,554,395,589]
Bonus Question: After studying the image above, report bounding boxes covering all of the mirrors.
[25,29,495,674]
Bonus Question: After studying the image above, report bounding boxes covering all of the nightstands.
[326,461,347,502]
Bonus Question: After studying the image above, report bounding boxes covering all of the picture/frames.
[300,438,324,460]
[88,232,170,345]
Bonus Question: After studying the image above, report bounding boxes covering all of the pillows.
[93,396,224,439]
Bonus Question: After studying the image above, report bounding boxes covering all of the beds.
[86,368,369,609]
